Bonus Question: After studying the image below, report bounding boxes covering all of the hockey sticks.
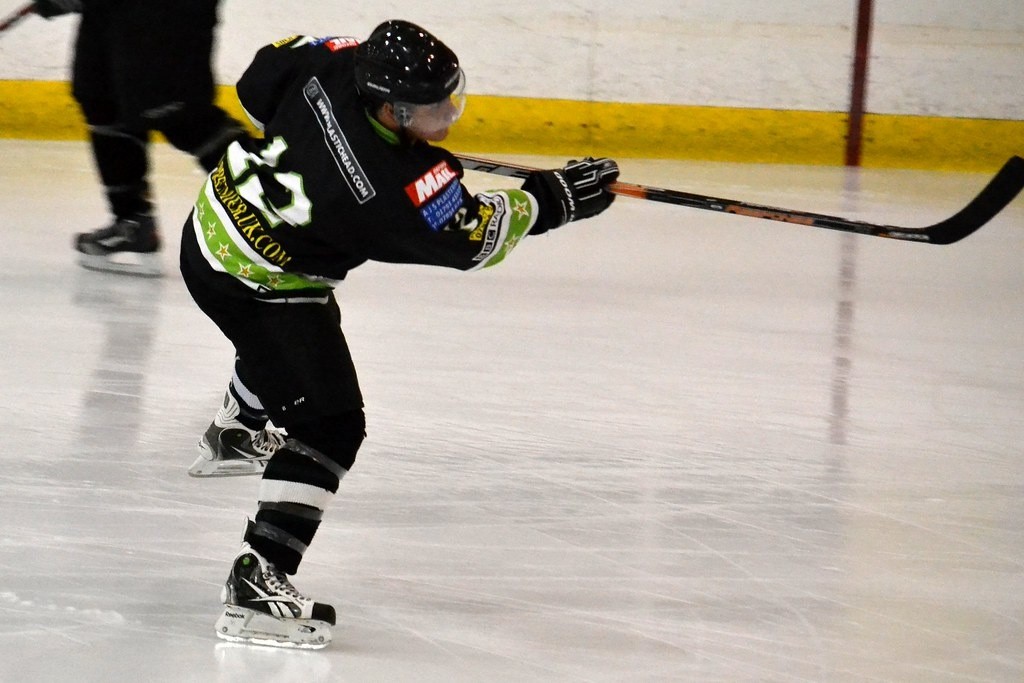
[456,152,1022,246]
[0,2,33,33]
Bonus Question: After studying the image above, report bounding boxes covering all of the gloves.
[31,0,69,18]
[521,157,620,235]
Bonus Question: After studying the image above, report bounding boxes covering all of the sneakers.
[188,384,287,478]
[73,213,168,278]
[215,517,336,650]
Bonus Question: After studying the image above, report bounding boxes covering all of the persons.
[180,18,619,651]
[1,1,268,278]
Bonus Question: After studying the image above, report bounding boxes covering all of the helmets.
[350,19,461,115]
